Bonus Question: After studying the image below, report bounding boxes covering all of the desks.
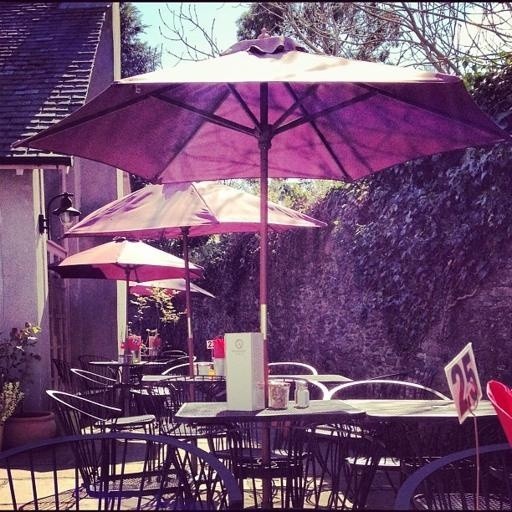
[176,399,366,510]
[141,375,226,401]
[119,355,184,359]
[268,375,354,384]
[89,361,167,417]
[341,399,497,425]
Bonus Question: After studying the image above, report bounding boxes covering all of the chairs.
[53,358,110,397]
[327,380,451,510]
[0,432,243,512]
[148,375,226,451]
[160,350,187,360]
[207,377,328,511]
[161,362,214,376]
[70,368,155,482]
[268,362,317,375]
[46,390,190,498]
[395,443,512,511]
[78,355,126,416]
[167,356,196,375]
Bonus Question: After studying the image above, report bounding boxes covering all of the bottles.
[269,383,291,409]
[125,337,133,365]
[143,345,146,354]
[296,382,309,408]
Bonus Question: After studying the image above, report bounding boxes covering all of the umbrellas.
[10,26,512,505]
[57,179,330,476]
[49,237,205,364]
[125,264,217,302]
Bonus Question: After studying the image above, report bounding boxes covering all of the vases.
[215,358,224,376]
[133,349,141,363]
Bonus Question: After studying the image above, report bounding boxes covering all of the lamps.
[39,192,81,236]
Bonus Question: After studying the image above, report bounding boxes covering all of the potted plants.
[0,322,56,453]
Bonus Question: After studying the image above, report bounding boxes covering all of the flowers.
[121,336,143,353]
[212,337,225,374]
[151,337,160,347]
